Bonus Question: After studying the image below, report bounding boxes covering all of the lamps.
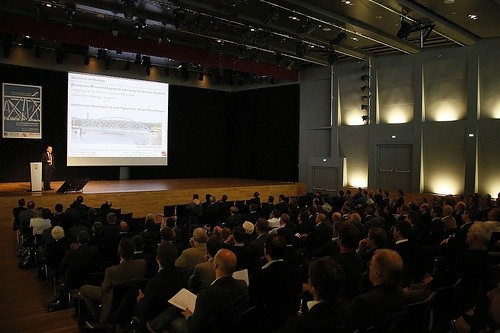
[327,50,338,66]
[330,31,348,48]
[396,18,412,42]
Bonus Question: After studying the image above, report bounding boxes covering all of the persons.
[12,187,500,333]
[40,145,54,191]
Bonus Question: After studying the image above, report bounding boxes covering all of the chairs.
[15,193,500,333]
